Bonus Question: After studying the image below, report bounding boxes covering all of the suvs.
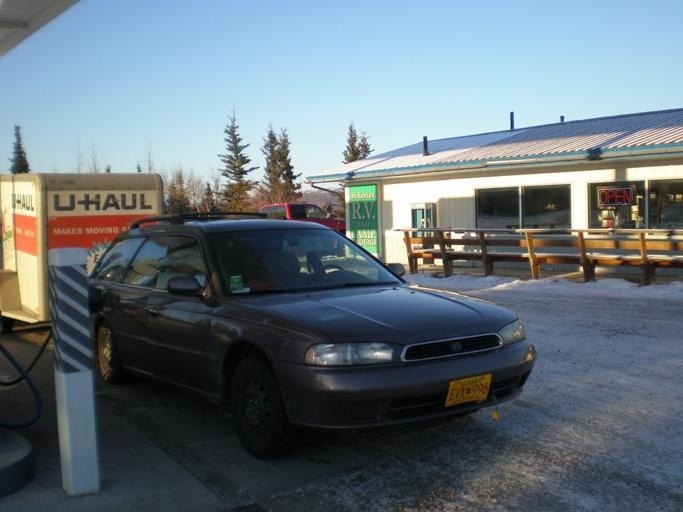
[257,203,346,235]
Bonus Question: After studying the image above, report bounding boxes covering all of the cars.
[88,211,536,460]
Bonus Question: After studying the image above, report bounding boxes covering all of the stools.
[402,227,683,291]
